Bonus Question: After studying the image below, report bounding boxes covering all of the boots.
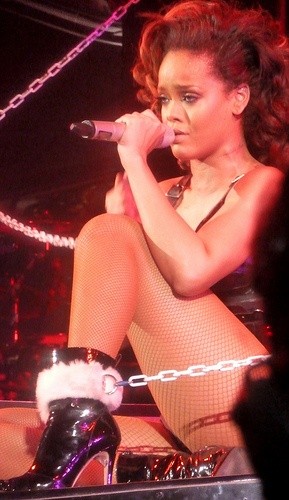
[113,444,253,484]
[0,347,123,496]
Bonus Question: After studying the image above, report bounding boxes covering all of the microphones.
[70,120,176,148]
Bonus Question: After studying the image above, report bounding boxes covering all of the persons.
[0,0,289,492]
